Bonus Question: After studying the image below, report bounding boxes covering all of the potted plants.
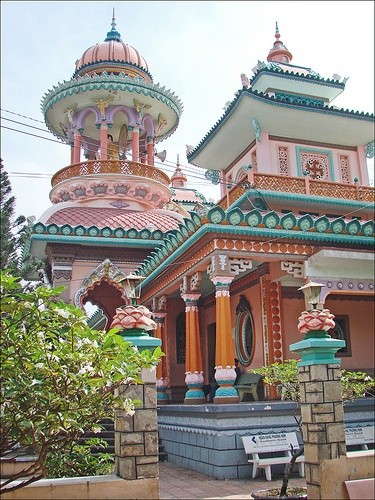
[248,359,311,500]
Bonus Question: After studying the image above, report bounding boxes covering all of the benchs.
[343,426,374,458]
[240,431,307,482]
[234,373,261,402]
